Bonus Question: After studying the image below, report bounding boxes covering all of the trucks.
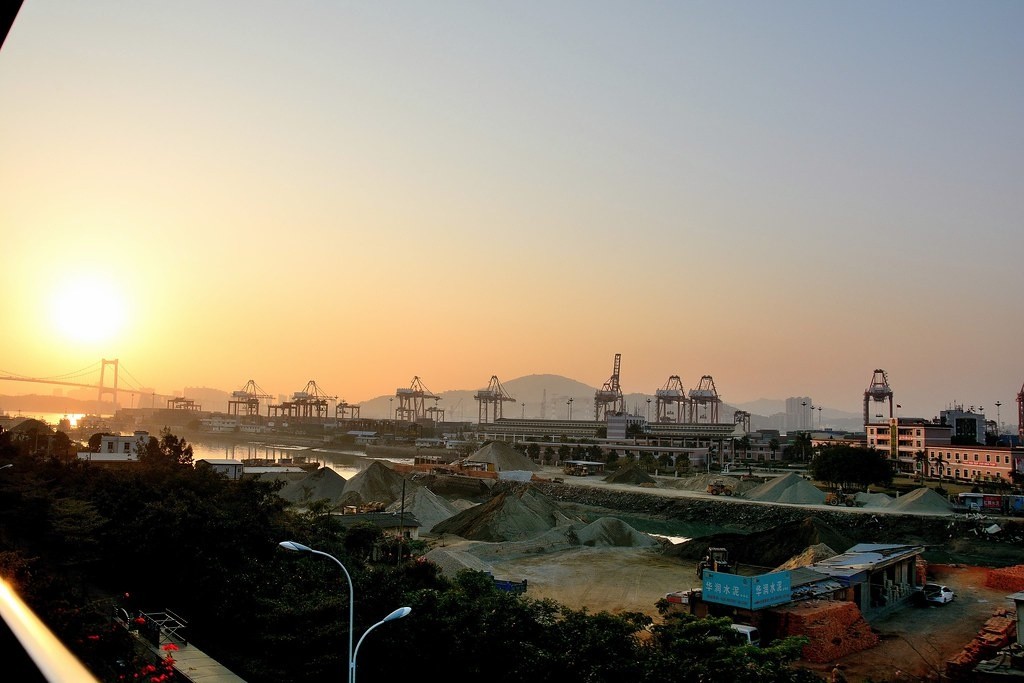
[953,502,982,514]
[478,571,528,596]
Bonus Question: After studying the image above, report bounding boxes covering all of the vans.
[730,623,762,648]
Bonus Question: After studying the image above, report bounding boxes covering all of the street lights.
[278,540,413,683]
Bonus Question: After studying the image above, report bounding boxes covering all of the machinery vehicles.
[824,489,868,507]
[707,479,741,496]
[563,464,589,476]
[695,546,731,581]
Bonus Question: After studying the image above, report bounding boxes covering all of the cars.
[921,583,955,606]
